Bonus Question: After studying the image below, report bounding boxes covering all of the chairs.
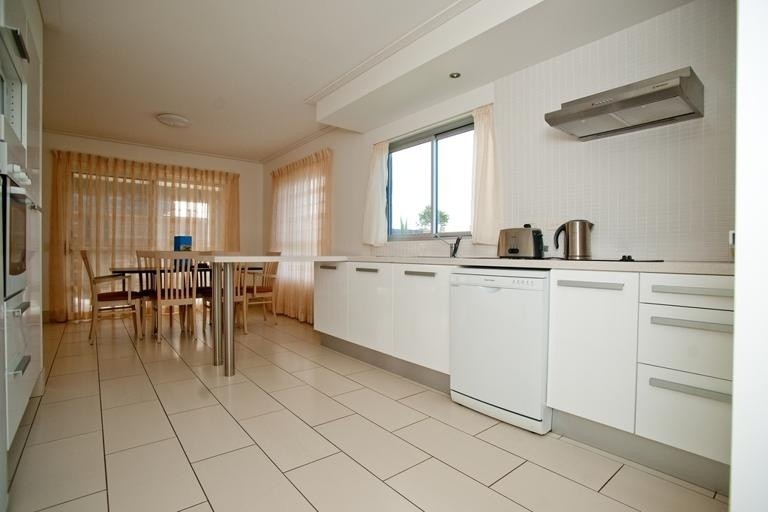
[79,250,282,346]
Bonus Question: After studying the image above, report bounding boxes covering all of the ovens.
[1,141,43,452]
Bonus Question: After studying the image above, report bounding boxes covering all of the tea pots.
[554,220,595,261]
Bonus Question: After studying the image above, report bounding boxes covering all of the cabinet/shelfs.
[4,283,44,452]
[547,270,639,495]
[314,261,450,395]
[641,274,735,498]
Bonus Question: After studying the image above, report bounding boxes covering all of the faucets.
[450,234,465,256]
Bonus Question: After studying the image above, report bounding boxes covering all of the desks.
[208,255,346,376]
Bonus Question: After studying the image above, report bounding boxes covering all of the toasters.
[497,224,544,259]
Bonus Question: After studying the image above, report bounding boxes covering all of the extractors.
[545,65,704,142]
